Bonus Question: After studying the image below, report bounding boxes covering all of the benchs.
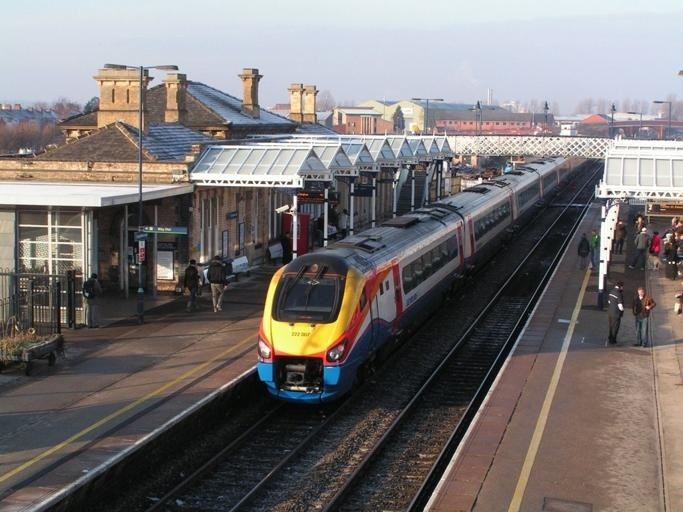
[268,243,284,266]
[201,255,259,293]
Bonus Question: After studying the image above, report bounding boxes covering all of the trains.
[256,157,587,405]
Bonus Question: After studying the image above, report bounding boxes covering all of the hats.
[190,260,196,264]
[91,273,97,279]
[214,256,220,260]
[653,231,659,234]
[642,227,647,232]
[615,280,624,286]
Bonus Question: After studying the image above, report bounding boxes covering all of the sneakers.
[214,306,222,312]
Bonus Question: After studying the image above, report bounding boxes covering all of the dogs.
[646,253,664,271]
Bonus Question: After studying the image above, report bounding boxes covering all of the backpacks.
[84,281,95,299]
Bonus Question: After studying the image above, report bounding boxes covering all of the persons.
[318,204,348,246]
[207,256,230,312]
[629,215,683,271]
[281,232,292,266]
[578,233,589,270]
[183,260,201,312]
[633,287,656,347]
[83,274,103,328]
[608,280,624,344]
[614,218,627,255]
[590,229,600,271]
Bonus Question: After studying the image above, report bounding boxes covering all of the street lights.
[542,101,549,132]
[468,100,482,136]
[103,63,178,324]
[411,98,444,135]
[609,100,672,140]
